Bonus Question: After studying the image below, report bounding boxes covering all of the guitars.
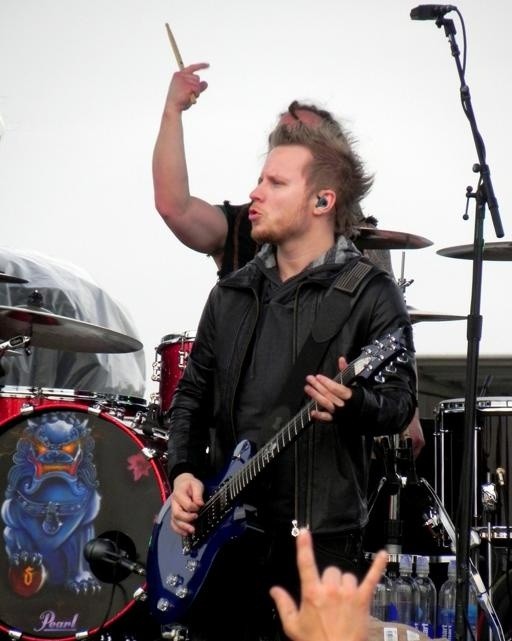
[144,328,408,627]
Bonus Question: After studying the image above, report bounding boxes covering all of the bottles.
[422,513,441,539]
[413,557,436,637]
[399,553,400,554]
[368,554,398,624]
[386,492,402,580]
[392,557,419,626]
[437,562,479,641]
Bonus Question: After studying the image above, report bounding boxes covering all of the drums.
[0,402,168,640]
[0,384,147,430]
[433,396,510,544]
[153,333,198,426]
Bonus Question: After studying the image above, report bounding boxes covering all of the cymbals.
[405,304,466,320]
[1,270,28,284]
[0,304,142,354]
[347,225,433,250]
[436,240,510,261]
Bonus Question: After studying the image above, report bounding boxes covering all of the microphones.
[83,537,146,579]
[409,2,458,24]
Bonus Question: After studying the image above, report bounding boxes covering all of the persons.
[167,119,419,640]
[152,63,427,456]
[268,528,389,641]
